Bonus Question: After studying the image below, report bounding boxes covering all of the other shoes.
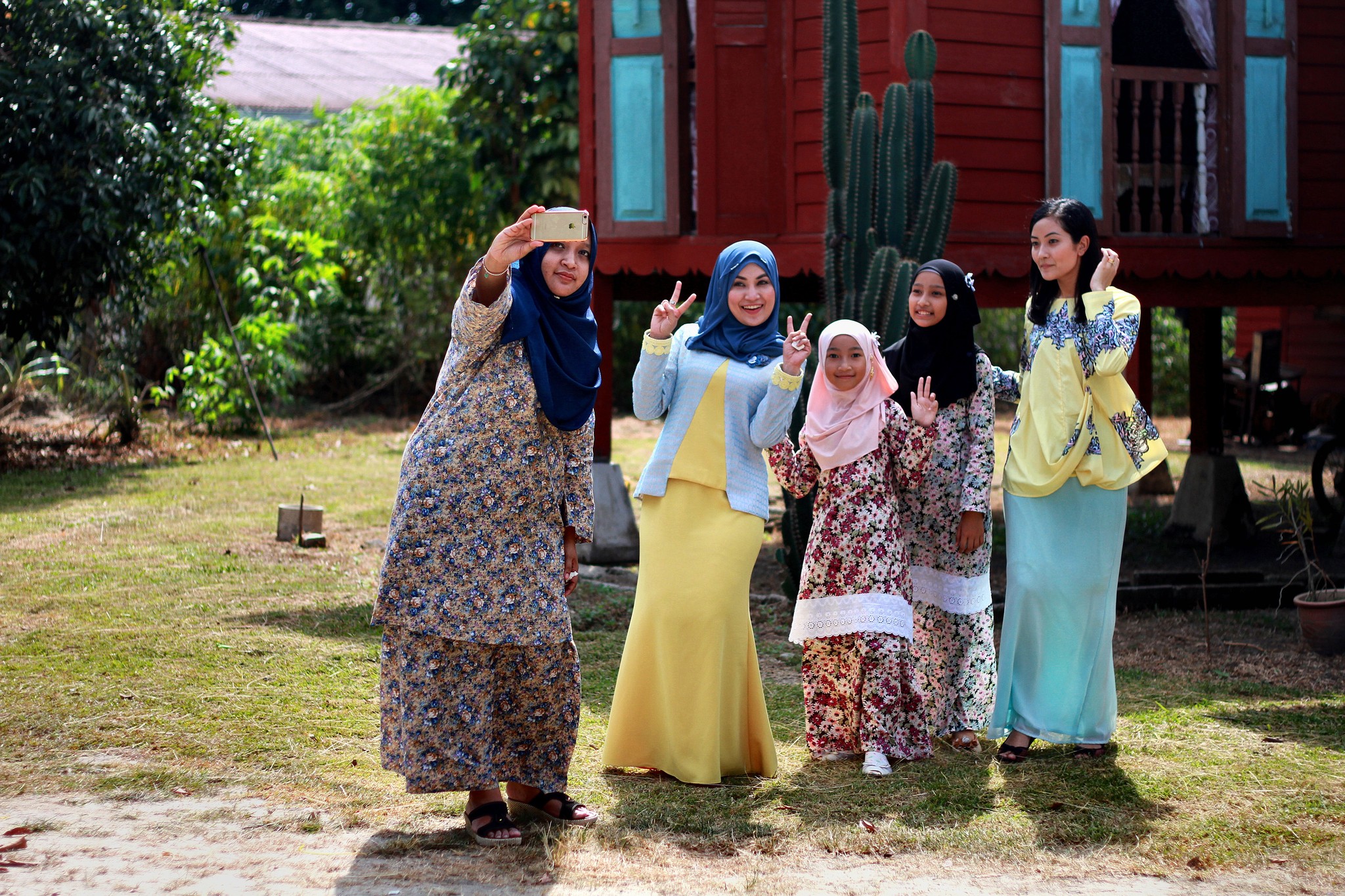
[1073,742,1105,759]
[996,726,1039,761]
[862,762,892,775]
[952,735,981,755]
[821,751,850,761]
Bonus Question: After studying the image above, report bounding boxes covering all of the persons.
[881,258,998,755]
[990,197,1169,763]
[763,317,939,776]
[369,205,602,846]
[601,240,813,783]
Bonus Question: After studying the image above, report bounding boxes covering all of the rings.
[1108,259,1115,264]
[669,299,677,306]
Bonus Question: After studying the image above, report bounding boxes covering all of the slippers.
[509,791,598,827]
[465,800,522,846]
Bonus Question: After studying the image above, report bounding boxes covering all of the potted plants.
[1250,470,1345,659]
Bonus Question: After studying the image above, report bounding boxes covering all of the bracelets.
[482,255,510,278]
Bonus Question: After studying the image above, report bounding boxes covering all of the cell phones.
[531,209,589,242]
